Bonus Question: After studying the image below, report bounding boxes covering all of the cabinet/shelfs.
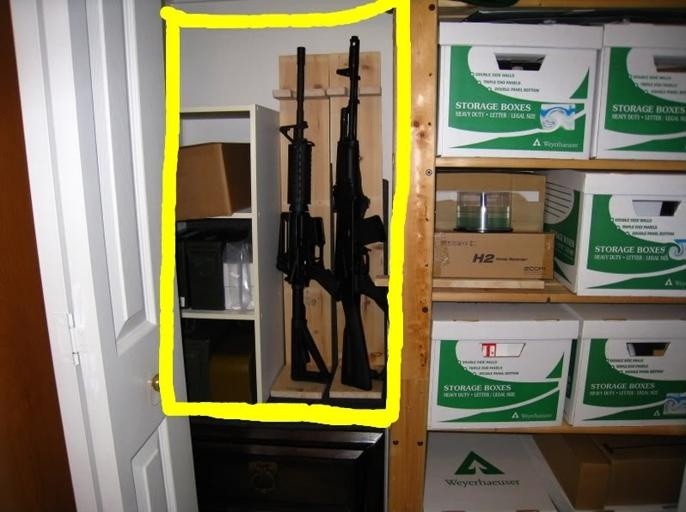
[179,103,285,403]
[387,0,686,512]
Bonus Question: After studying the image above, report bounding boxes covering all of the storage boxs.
[174,227,248,310]
[175,141,250,221]
[419,19,686,512]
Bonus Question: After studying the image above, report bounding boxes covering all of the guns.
[277,47,333,384]
[334,36,388,390]
